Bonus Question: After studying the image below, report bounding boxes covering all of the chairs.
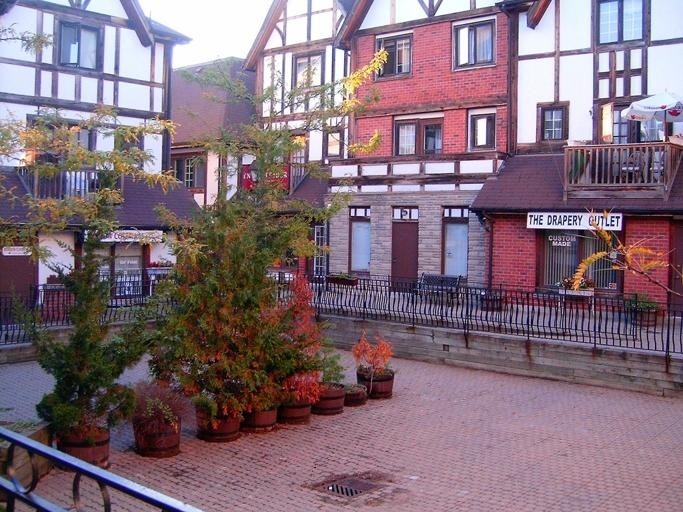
[622,151,646,183]
[650,153,663,183]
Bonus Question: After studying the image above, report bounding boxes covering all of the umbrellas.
[617,86,682,142]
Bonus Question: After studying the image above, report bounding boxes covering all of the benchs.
[411,272,462,307]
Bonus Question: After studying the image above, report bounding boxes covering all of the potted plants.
[8,174,395,471]
[624,289,658,326]
